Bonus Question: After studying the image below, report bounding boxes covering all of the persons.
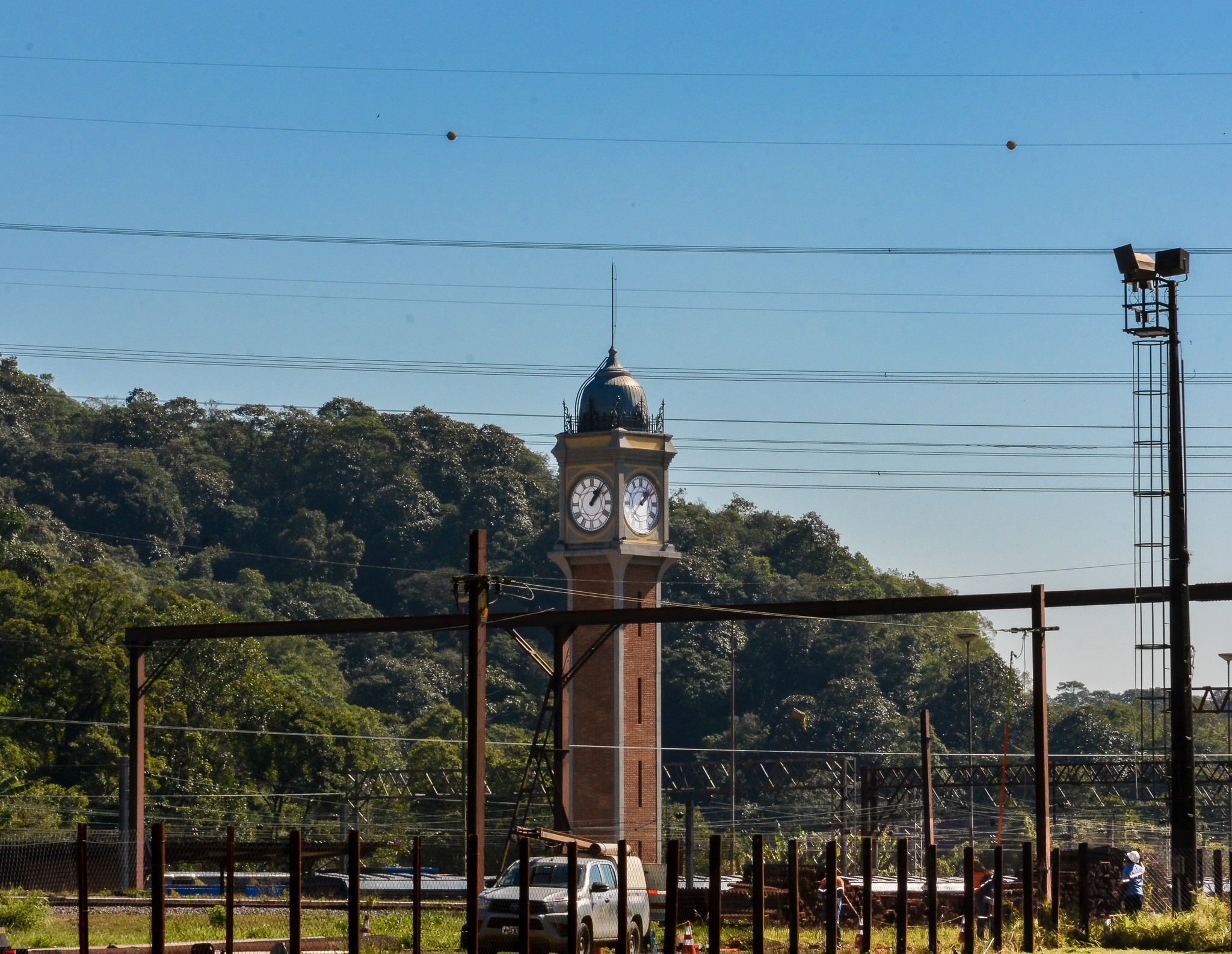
[973,860,1012,942]
[817,874,845,947]
[1118,850,1145,922]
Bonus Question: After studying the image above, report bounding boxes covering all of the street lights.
[1113,242,1192,843]
[955,633,981,847]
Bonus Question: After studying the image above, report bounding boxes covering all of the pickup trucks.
[477,855,650,954]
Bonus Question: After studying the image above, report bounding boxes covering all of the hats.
[1126,851,1140,863]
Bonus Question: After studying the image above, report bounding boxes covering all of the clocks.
[567,473,616,536]
[623,473,663,537]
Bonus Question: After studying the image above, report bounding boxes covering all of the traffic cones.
[593,946,603,954]
[1102,915,1112,938]
[854,916,864,947]
[681,921,696,954]
[361,915,371,937]
[958,917,965,944]
